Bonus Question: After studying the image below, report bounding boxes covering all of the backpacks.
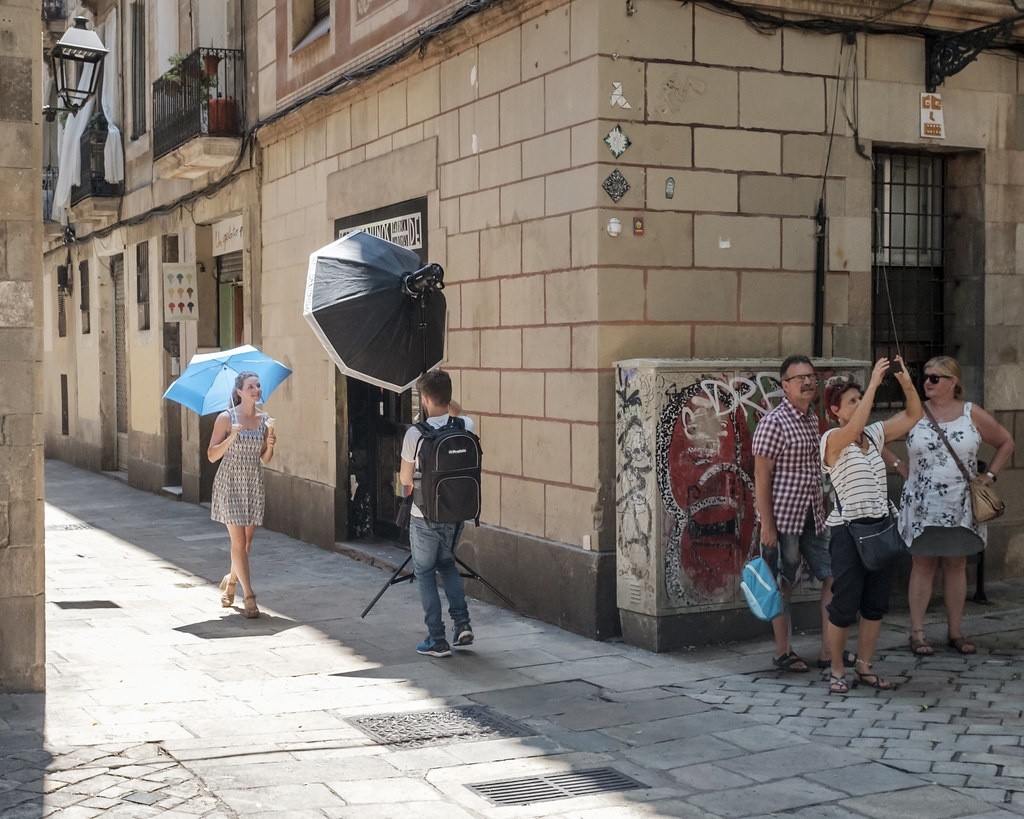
[413,416,483,524]
[741,541,790,622]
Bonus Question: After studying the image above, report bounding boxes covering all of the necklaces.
[855,434,863,445]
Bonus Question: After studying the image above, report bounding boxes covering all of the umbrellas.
[301,230,447,395]
[161,344,295,424]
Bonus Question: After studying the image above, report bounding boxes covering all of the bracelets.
[986,471,997,482]
[893,457,904,467]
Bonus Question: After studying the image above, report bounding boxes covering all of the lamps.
[42,17,110,122]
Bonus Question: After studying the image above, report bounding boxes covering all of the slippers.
[773,651,808,672]
[818,651,856,666]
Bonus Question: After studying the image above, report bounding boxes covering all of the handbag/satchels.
[969,480,1005,521]
[848,512,906,570]
[396,495,412,529]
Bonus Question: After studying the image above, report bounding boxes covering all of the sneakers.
[452,623,474,646]
[417,639,452,657]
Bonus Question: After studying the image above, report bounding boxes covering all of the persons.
[880,357,1016,654]
[818,358,924,693]
[207,371,277,618]
[752,356,858,671]
[400,369,475,658]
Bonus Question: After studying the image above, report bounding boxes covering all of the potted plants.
[156,46,222,106]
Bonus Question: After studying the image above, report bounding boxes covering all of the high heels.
[243,594,259,618]
[219,575,237,606]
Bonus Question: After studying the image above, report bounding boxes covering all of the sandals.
[829,672,848,692]
[948,634,976,654]
[909,636,933,655]
[854,669,895,689]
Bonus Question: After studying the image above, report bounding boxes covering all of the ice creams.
[265,417,276,434]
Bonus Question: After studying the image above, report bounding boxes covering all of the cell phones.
[886,361,903,373]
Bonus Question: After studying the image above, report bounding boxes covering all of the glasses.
[785,373,816,382]
[923,374,953,384]
[830,382,848,398]
